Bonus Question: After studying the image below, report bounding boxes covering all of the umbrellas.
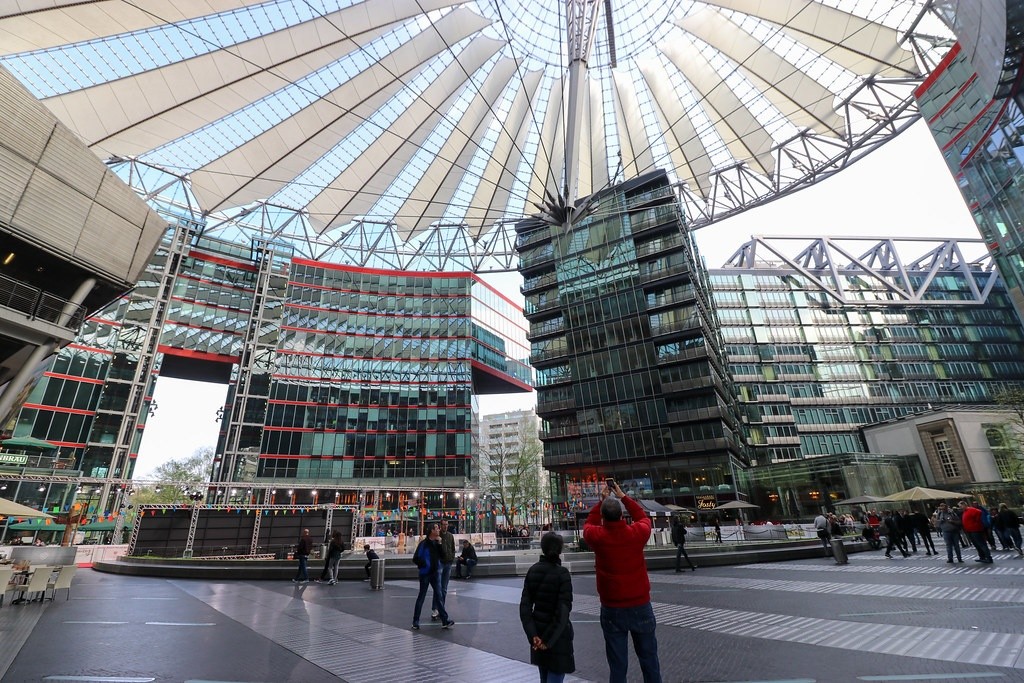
[883,485,972,518]
[664,505,686,511]
[830,495,892,512]
[711,500,759,509]
[0,497,57,519]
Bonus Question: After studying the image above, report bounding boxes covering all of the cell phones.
[606,478,616,491]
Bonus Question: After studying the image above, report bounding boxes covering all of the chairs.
[0,564,77,608]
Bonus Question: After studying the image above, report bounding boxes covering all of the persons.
[519,532,576,683]
[314,530,345,584]
[412,523,455,630]
[715,518,723,543]
[583,482,663,683]
[430,519,455,619]
[456,540,478,580]
[292,529,312,583]
[671,515,698,573]
[363,545,379,582]
[813,501,1024,570]
[377,524,547,551]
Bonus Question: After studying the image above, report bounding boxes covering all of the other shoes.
[412,624,419,630]
[884,553,893,558]
[327,579,335,585]
[302,580,309,582]
[1016,547,1024,555]
[947,561,953,564]
[715,540,718,543]
[442,621,454,628]
[934,552,938,554]
[691,566,697,571]
[1003,547,1015,551]
[292,578,298,583]
[926,552,931,555]
[991,546,996,551]
[456,574,461,579]
[976,559,993,563]
[904,553,911,558]
[364,578,370,581]
[676,569,685,573]
[958,561,964,563]
[961,544,967,548]
[719,540,722,543]
[314,579,322,584]
[431,610,439,618]
[466,576,470,579]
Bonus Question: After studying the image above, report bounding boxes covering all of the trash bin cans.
[830,539,848,563]
[370,559,385,589]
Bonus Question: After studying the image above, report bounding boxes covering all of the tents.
[624,500,674,528]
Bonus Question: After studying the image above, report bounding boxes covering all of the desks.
[9,567,62,605]
